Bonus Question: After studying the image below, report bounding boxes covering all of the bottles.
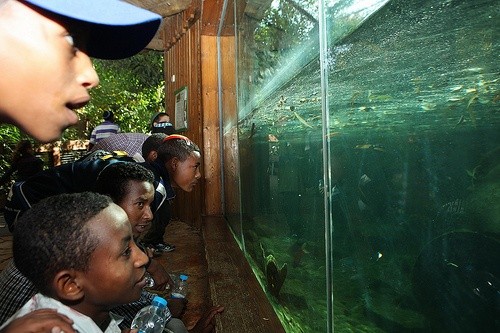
[171,275,189,299]
[131,296,167,333]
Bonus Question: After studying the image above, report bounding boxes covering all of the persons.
[0,0,162,333]
[0,192,149,333]
[0,112,225,333]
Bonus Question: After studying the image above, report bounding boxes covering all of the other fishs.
[246,68,500,153]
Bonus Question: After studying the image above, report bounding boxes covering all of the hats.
[21,0,162,60]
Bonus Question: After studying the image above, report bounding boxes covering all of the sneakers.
[155,242,176,254]
[140,243,162,256]
